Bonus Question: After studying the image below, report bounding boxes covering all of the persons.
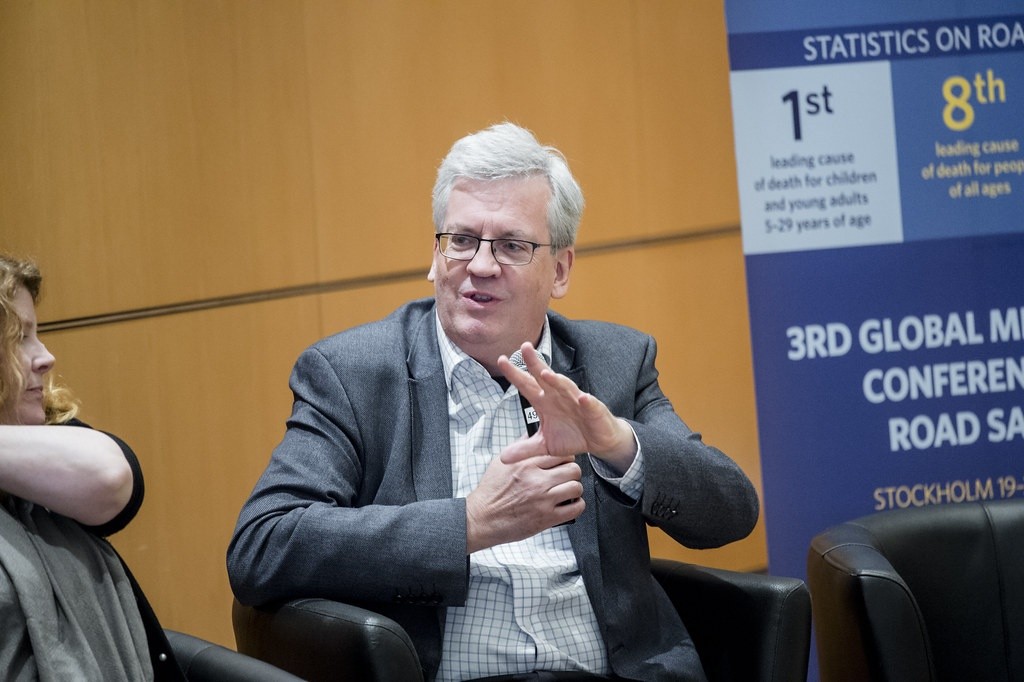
[1,250,182,682]
[227,121,759,682]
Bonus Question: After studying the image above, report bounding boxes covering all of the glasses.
[437,232,553,265]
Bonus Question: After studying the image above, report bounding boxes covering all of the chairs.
[163,557,812,682]
[807,498,1024,682]
